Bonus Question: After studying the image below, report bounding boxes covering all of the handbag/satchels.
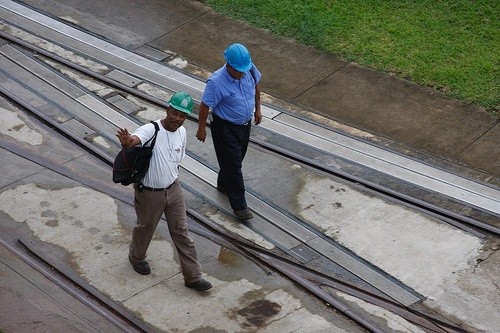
[113,121,160,186]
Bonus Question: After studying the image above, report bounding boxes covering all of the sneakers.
[184,279,212,290]
[129,256,151,274]
[217,183,224,190]
[234,208,253,219]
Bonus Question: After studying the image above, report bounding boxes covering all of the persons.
[196,43,262,220]
[116,91,213,291]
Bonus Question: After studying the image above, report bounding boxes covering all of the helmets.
[224,43,252,72]
[169,92,193,114]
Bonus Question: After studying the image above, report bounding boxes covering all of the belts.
[213,115,251,128]
[140,181,175,191]
